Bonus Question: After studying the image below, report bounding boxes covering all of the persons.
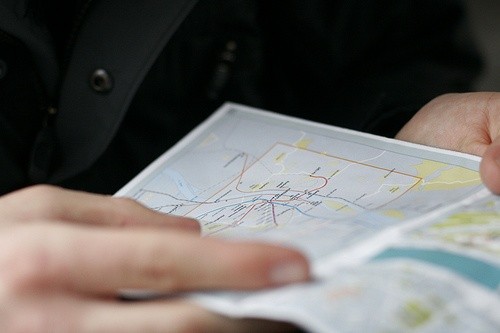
[0,1,500,333]
